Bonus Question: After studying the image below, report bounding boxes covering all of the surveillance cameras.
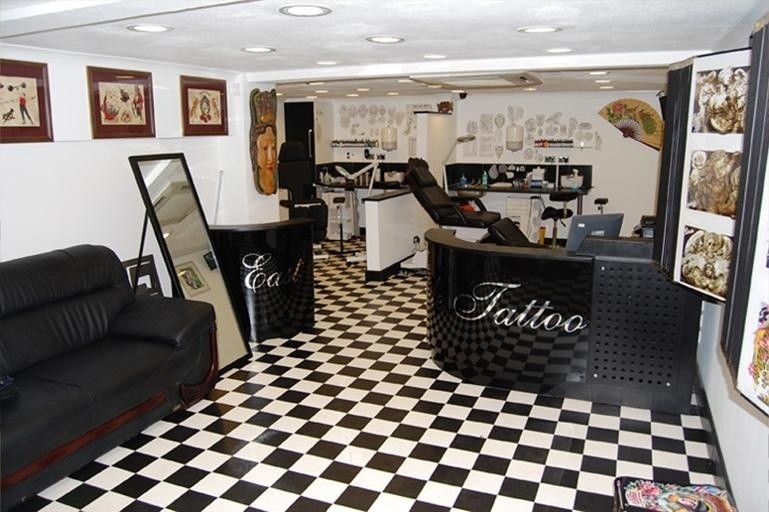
[459,93,467,99]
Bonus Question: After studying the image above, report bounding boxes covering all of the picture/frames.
[86,66,156,139]
[1,58,54,144]
[179,75,229,137]
[174,263,208,295]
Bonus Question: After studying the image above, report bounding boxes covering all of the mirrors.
[129,154,252,375]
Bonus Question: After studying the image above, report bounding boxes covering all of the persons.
[681,66,749,300]
[625,479,711,511]
[15,88,37,125]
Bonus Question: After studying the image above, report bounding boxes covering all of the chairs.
[402,157,529,248]
[542,191,577,246]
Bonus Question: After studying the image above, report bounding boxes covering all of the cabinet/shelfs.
[319,190,359,241]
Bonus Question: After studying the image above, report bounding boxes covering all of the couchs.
[2,245,220,511]
